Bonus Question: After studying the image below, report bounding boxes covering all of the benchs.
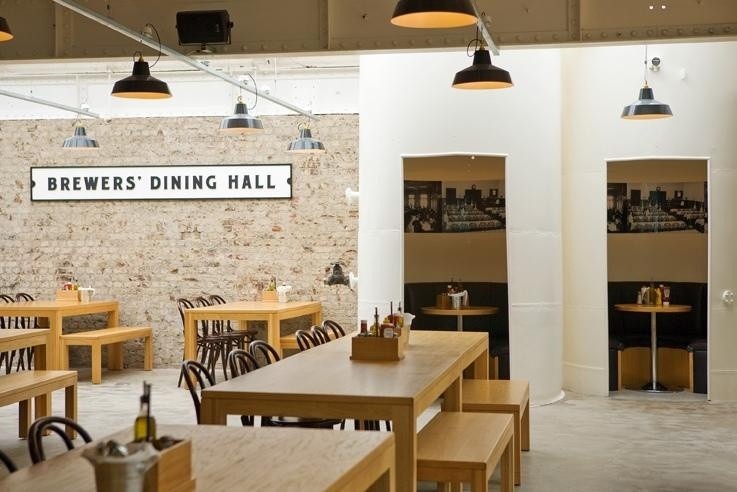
[59,326,152,384]
[1,368,77,439]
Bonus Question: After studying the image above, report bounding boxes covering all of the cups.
[78,287,95,304]
[263,290,276,301]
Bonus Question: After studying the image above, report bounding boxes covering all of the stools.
[442,378,530,487]
[417,411,517,491]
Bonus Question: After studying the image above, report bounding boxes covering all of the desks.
[2,299,119,372]
[2,424,395,492]
[199,326,490,492]
[419,303,501,331]
[612,303,692,395]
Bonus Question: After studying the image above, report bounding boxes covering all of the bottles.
[278,283,289,303]
[82,440,161,492]
[356,301,403,338]
[269,279,274,292]
[135,394,157,443]
[446,284,465,295]
[63,278,81,291]
[635,284,671,308]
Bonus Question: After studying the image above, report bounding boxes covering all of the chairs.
[179,295,394,434]
[1,450,19,474]
[0,291,54,374]
[26,415,94,463]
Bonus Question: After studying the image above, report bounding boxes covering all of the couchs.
[607,281,708,395]
[404,281,510,381]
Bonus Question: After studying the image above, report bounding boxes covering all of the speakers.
[176,10,230,44]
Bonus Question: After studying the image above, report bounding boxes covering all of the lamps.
[0,0,677,157]
